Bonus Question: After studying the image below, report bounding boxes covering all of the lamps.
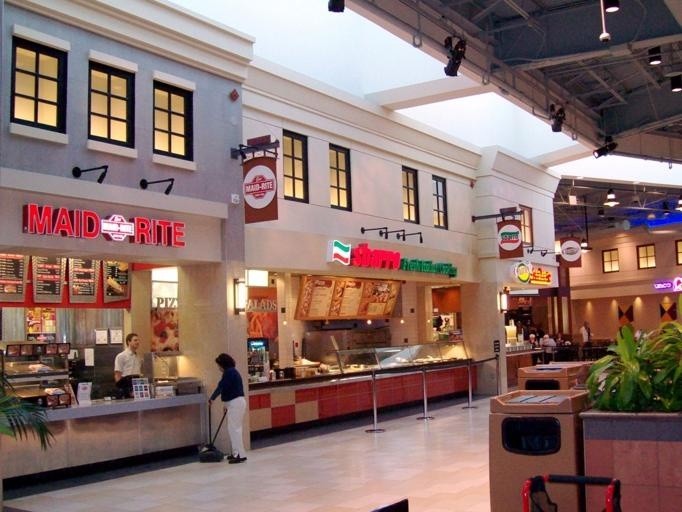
[234,277,247,314]
[238,144,277,159]
[140,179,175,194]
[549,106,570,134]
[605,2,682,93]
[555,179,682,256]
[72,165,108,183]
[361,228,424,243]
[593,139,621,159]
[437,34,470,79]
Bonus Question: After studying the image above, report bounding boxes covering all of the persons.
[527,320,594,367]
[114,333,144,382]
[208,353,247,464]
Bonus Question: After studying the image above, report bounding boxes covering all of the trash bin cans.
[490,360,599,511]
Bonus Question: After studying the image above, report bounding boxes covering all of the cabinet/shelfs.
[0,350,72,412]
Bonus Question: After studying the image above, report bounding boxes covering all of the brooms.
[201,401,224,451]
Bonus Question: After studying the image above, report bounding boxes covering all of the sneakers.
[227,454,246,464]
